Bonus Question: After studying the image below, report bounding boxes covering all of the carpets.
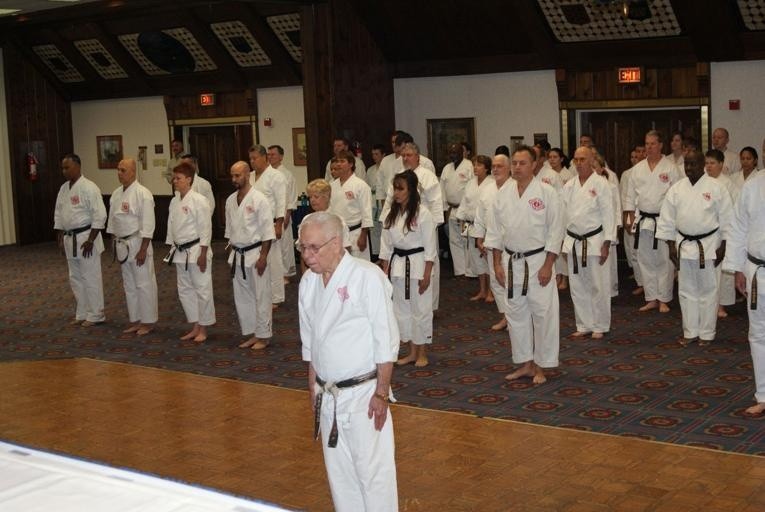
[1,236,765,458]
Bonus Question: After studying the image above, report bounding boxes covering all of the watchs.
[373,390,392,403]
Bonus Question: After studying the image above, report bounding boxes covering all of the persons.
[51,153,108,332]
[377,169,438,368]
[161,126,765,346]
[483,145,568,385]
[222,161,277,350]
[293,209,404,510]
[720,167,765,415]
[105,157,159,337]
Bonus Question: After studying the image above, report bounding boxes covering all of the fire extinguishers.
[355,141,363,160]
[27,151,39,181]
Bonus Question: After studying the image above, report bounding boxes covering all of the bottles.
[300,191,307,208]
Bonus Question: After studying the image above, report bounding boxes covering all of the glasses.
[296,237,335,253]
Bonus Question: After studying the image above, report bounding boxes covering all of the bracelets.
[87,238,94,244]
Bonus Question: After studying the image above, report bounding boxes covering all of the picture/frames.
[96,135,123,169]
[427,117,477,177]
[292,128,307,166]
